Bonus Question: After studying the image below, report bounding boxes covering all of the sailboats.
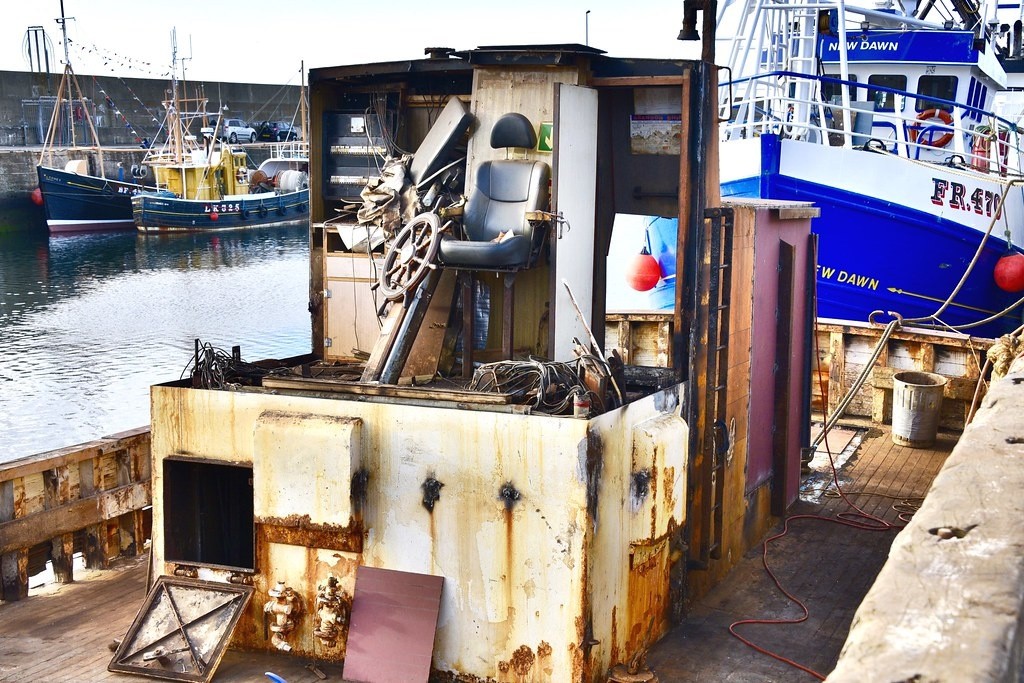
[36,0,308,234]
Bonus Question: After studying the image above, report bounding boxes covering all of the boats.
[715,0,1024,338]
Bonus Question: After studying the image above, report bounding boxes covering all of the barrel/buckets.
[970,129,1010,178]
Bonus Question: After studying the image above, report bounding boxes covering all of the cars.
[208,118,258,144]
[259,121,297,141]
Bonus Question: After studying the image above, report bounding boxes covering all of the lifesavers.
[909,108,955,151]
